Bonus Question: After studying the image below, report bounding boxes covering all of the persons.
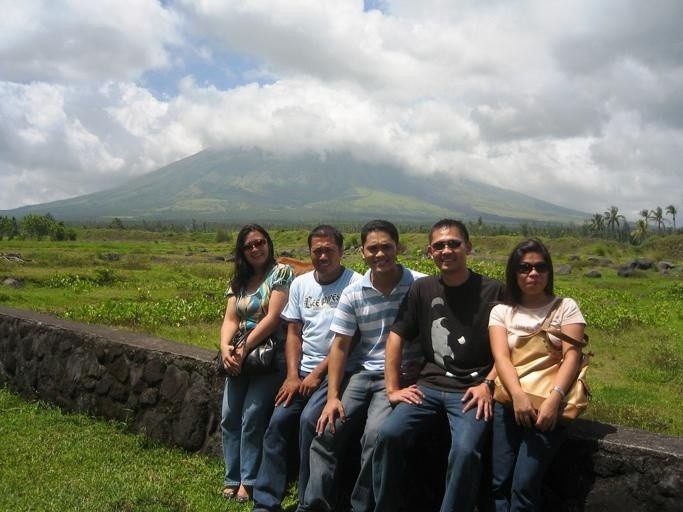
[213,223,295,503]
[369,218,508,511]
[250,224,363,511]
[296,219,432,511]
[487,235,584,510]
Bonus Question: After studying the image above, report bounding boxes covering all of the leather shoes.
[236,493,251,504]
[222,489,235,498]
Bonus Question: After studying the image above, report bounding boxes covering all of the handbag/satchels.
[215,328,280,378]
[492,297,593,422]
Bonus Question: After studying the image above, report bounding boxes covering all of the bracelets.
[550,387,564,397]
[239,340,250,349]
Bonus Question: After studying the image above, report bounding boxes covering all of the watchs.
[482,378,495,394]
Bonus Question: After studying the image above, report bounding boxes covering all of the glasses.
[429,240,464,251]
[239,238,266,251]
[514,262,550,275]
